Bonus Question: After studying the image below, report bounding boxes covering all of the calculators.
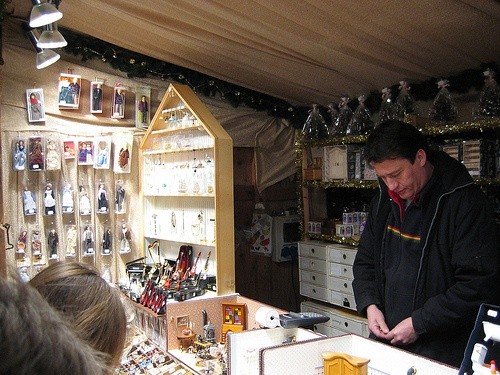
[279,311,330,329]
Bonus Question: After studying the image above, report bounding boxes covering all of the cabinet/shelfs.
[321,351,370,375]
[298,126,500,240]
[220,303,246,344]
[300,301,371,338]
[138,83,235,295]
[297,241,358,311]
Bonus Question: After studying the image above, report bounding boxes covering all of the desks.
[177,333,196,347]
[194,340,213,354]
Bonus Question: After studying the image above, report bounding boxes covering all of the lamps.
[36,23,68,48]
[22,21,61,69]
[29,0,63,28]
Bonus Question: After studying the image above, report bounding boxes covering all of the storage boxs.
[336,212,368,237]
[308,221,321,233]
[304,139,500,182]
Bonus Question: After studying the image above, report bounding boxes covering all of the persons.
[119,224,131,250]
[15,140,107,171]
[84,226,94,253]
[352,118,500,370]
[29,261,126,375]
[98,185,108,212]
[31,94,42,120]
[63,184,73,210]
[23,183,60,215]
[32,231,41,253]
[18,231,26,251]
[116,185,126,212]
[79,186,91,213]
[0,267,102,375]
[139,95,147,123]
[68,226,77,255]
[94,84,102,110]
[68,76,80,105]
[103,228,111,254]
[48,230,58,258]
[116,89,123,115]
